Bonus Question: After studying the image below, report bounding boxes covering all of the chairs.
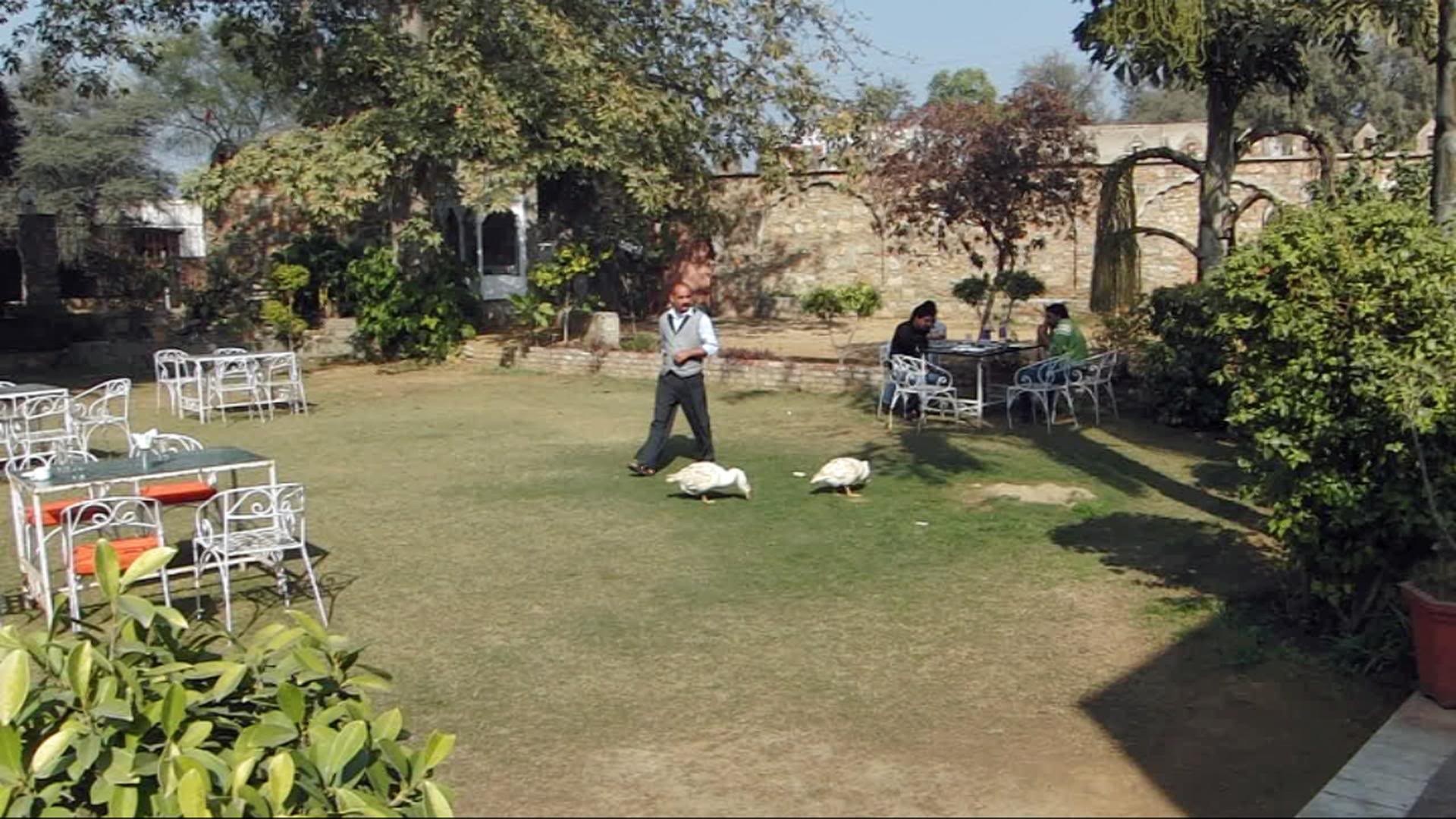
[0,339,332,636]
[875,321,964,429]
[1004,347,1130,432]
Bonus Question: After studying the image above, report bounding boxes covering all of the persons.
[626,284,718,477]
[1015,304,1089,425]
[883,300,947,420]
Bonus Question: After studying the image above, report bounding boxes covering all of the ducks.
[809,457,871,497]
[665,461,752,503]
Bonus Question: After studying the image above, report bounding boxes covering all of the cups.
[31,467,50,482]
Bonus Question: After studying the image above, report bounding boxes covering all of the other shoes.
[628,462,656,476]
[1053,413,1073,424]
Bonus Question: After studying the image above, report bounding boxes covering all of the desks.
[934,338,1044,419]
[0,384,70,471]
[178,348,290,422]
[916,342,1018,428]
[11,447,282,633]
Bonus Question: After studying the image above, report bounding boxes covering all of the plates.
[20,472,34,478]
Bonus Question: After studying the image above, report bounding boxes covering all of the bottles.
[982,321,991,345]
[998,318,1007,344]
